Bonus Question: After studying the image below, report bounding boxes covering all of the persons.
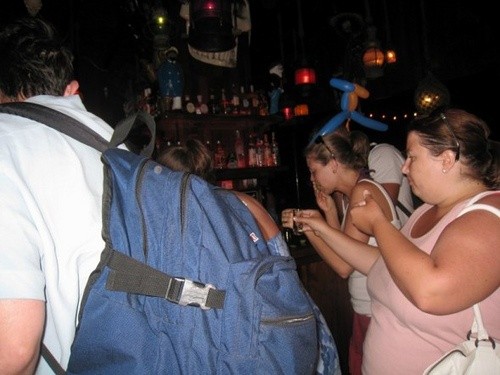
[0,15,344,375]
[275,108,499,375]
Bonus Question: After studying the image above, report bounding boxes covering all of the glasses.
[314,135,332,153]
[430,105,461,162]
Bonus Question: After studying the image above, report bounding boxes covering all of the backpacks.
[0,100,320,375]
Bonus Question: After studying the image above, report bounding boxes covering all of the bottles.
[207,131,280,169]
[185,85,259,114]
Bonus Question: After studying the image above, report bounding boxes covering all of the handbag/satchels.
[423,337,500,375]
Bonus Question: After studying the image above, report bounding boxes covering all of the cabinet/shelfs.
[143,112,307,251]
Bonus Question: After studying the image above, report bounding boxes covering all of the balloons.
[307,77,388,146]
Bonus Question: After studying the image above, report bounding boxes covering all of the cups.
[292,208,304,236]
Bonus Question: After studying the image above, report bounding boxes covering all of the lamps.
[360,0,386,78]
[186,0,236,53]
[413,8,450,118]
